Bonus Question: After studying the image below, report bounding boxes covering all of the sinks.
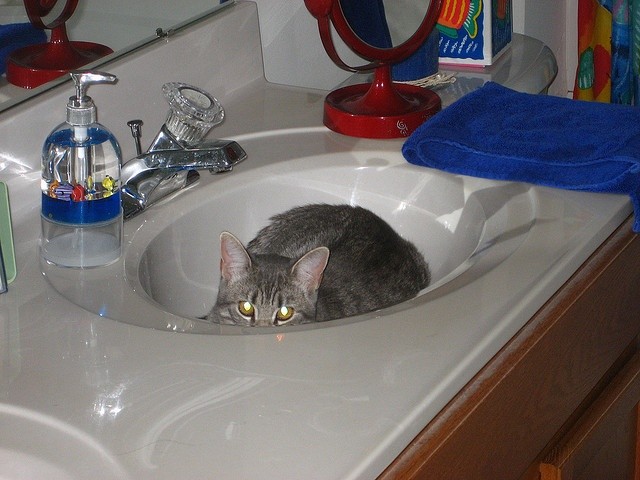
[121,148,540,334]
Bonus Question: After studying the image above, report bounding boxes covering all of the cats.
[196,202,431,327]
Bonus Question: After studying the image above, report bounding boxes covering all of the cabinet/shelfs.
[380,218,639,479]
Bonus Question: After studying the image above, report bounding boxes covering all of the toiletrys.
[33,68,124,270]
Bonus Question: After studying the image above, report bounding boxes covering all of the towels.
[401,80,640,238]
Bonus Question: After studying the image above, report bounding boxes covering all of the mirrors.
[0,0,237,116]
[329,1,443,59]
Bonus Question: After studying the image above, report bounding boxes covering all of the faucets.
[120,142,248,217]
